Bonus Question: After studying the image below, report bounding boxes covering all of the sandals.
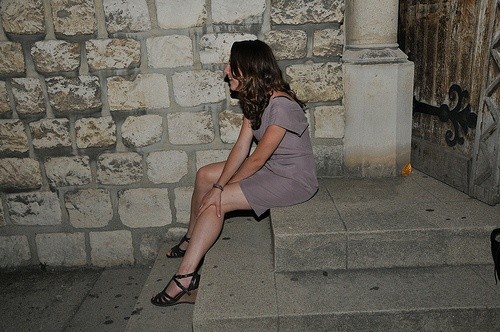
[166,233,190,258]
[150,271,201,306]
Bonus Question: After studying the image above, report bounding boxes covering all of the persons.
[150,38,320,308]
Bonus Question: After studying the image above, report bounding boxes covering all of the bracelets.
[212,183,224,191]
